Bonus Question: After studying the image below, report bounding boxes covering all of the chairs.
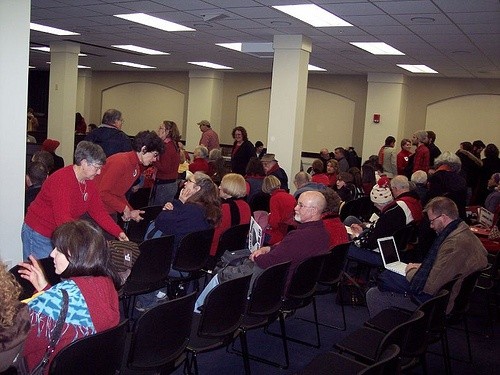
[7,185,500,375]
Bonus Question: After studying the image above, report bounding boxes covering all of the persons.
[17,220,121,375]
[89,108,133,157]
[149,120,183,206]
[25,107,99,209]
[185,130,500,293]
[22,140,129,264]
[230,126,258,177]
[210,173,251,259]
[366,196,488,319]
[81,129,165,242]
[196,190,330,312]
[0,262,29,375]
[142,170,223,308]
[198,120,220,157]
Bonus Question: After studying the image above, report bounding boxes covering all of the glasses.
[295,203,314,208]
[188,179,196,184]
[428,215,441,224]
[151,151,158,158]
[90,163,102,171]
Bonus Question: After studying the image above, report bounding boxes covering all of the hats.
[197,120,210,126]
[370,176,392,203]
[413,130,429,144]
[260,154,278,162]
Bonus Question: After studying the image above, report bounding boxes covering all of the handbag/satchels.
[377,269,410,295]
[17,289,69,375]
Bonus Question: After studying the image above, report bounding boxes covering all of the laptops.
[376,236,408,276]
